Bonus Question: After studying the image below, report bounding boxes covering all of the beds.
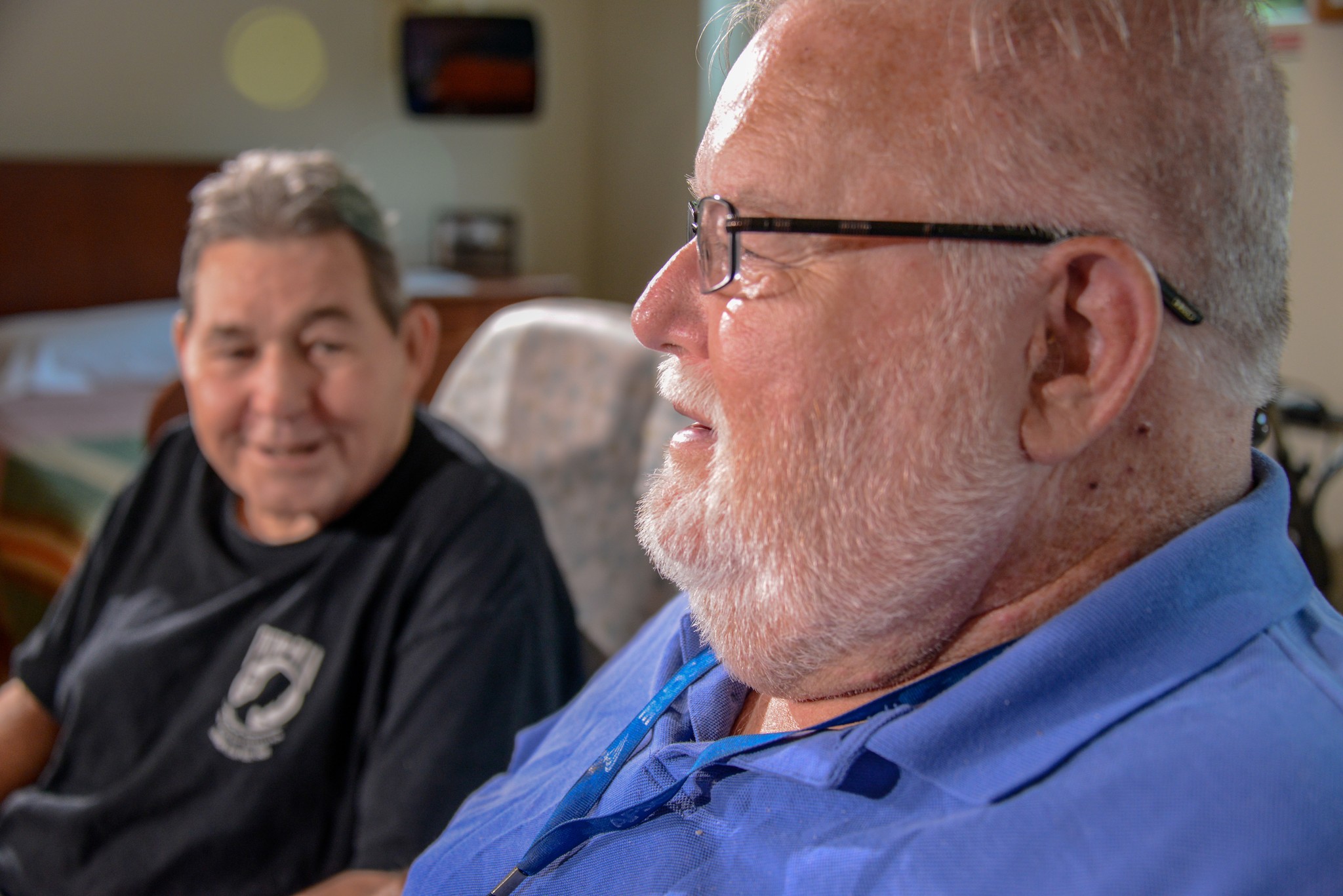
[0,159,225,636]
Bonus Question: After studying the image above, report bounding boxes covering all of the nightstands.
[407,275,576,402]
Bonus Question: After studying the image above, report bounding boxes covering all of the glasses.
[684,195,1204,346]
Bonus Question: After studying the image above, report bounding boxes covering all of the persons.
[385,1,1343,896]
[0,142,592,896]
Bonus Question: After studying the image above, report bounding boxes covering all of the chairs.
[430,295,681,700]
